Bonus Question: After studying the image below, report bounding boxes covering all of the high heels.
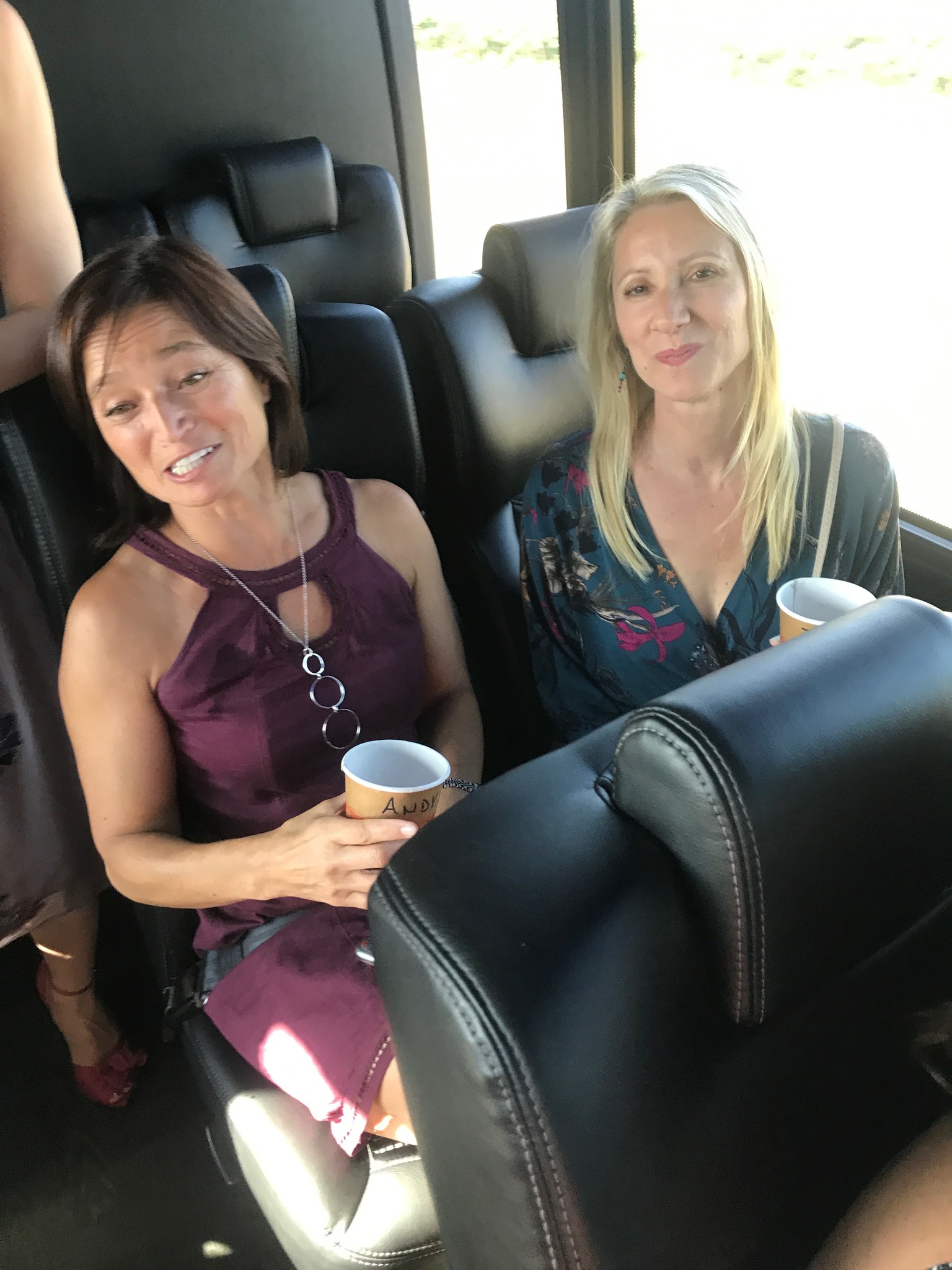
[33,958,146,1108]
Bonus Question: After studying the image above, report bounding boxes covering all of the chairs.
[385,203,594,696]
[366,594,952,1270]
[0,265,449,1269]
[169,135,417,304]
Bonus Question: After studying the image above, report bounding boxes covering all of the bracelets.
[443,779,482,793]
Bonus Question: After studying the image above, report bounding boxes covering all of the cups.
[341,739,449,832]
[776,579,874,644]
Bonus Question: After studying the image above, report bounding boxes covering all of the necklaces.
[174,478,360,750]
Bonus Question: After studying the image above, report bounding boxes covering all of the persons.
[0,0,138,1109]
[520,167,903,748]
[47,237,490,1159]
